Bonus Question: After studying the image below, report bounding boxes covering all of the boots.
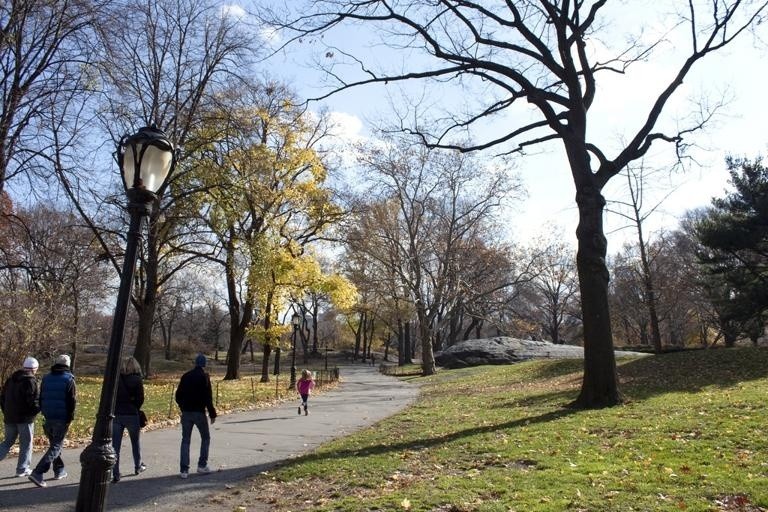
[298,404,304,414]
[305,409,311,416]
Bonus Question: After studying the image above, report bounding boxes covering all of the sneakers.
[54,473,67,479]
[197,466,211,473]
[28,475,47,487]
[16,469,32,476]
[113,474,120,481]
[181,471,188,478]
[135,463,147,474]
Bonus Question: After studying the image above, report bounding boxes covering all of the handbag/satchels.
[139,410,147,427]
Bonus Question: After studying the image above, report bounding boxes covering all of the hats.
[195,355,206,367]
[23,356,39,371]
[55,354,71,367]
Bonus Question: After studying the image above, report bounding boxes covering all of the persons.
[295,368,316,416]
[174,353,218,480]
[108,355,148,483]
[27,354,78,489]
[0,356,41,478]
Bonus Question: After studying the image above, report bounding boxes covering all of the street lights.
[75,122,180,512]
[351,343,354,364]
[289,311,301,391]
[325,342,328,370]
